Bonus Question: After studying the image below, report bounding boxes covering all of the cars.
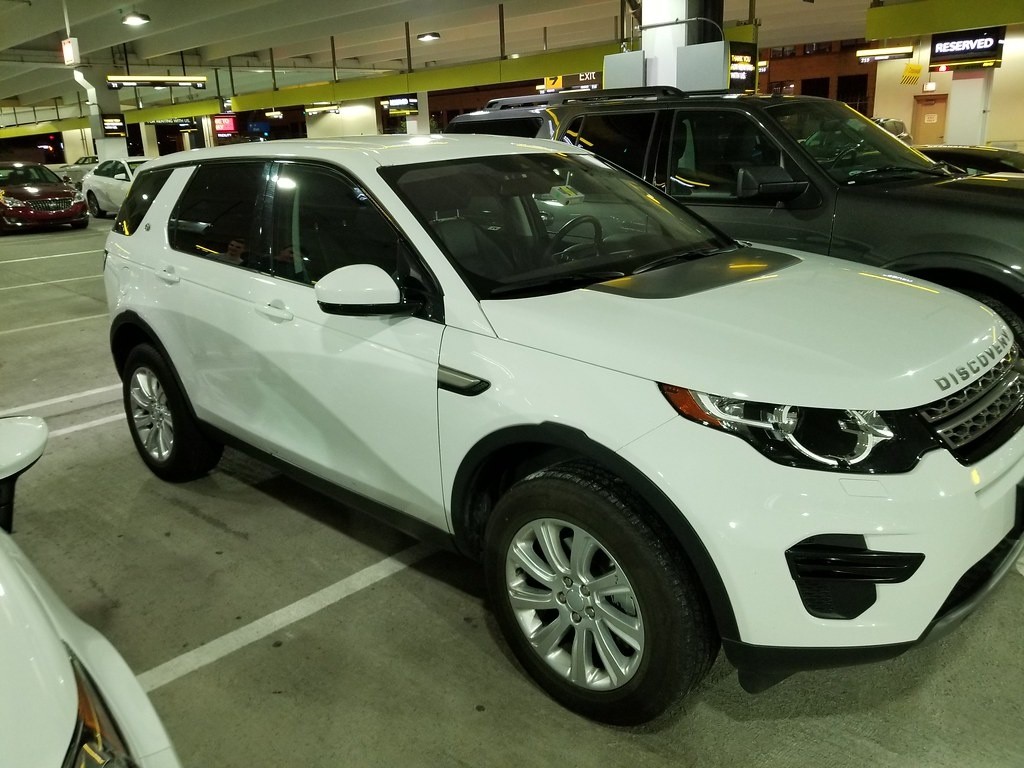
[1,416,181,768]
[912,144,1022,170]
[59,156,99,171]
[0,161,89,234]
[82,157,153,218]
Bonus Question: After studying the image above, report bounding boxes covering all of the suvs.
[446,88,1023,357]
[102,133,1024,725]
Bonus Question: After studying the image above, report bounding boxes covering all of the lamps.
[120,4,150,26]
[416,31,441,41]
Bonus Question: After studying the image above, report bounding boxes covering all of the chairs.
[430,216,513,280]
